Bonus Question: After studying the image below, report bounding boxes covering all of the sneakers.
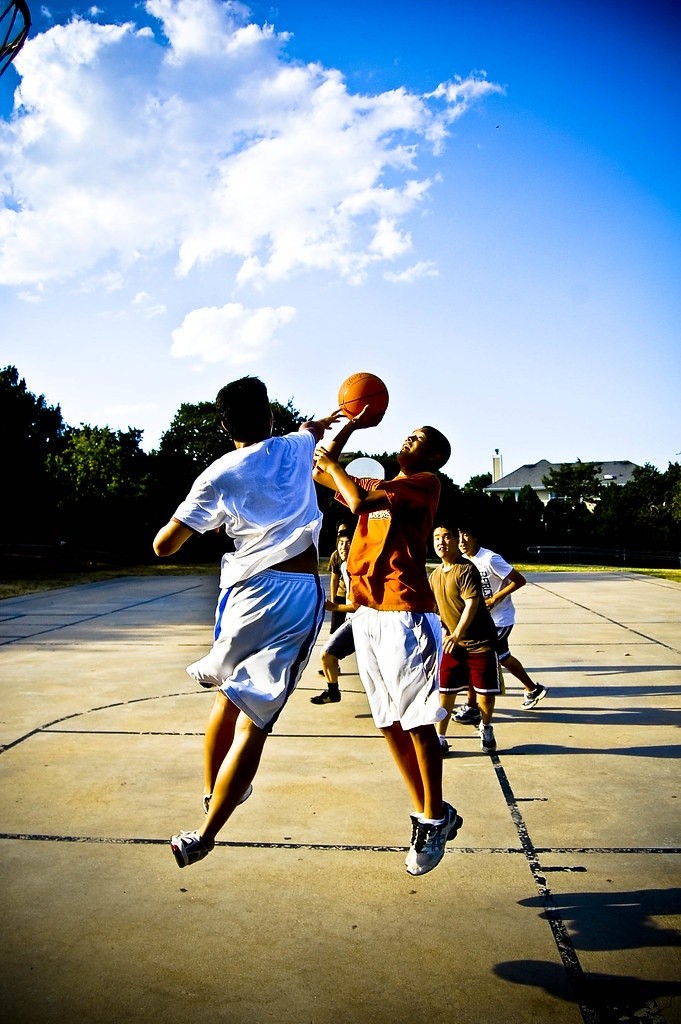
[319,666,340,676]
[310,689,341,705]
[452,703,480,723]
[479,720,497,753]
[203,784,253,814]
[521,682,549,710]
[440,740,452,752]
[170,830,214,869]
[404,801,463,876]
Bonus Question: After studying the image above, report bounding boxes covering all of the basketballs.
[337,371,389,423]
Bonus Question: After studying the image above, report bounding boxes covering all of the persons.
[451,522,550,724]
[312,404,462,875]
[318,519,350,676]
[310,529,353,705]
[428,522,506,754]
[152,376,348,867]
